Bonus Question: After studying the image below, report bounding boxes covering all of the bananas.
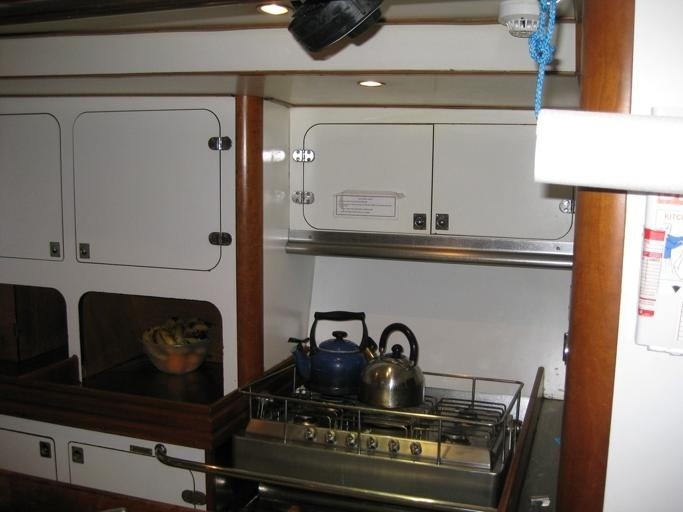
[144,325,203,358]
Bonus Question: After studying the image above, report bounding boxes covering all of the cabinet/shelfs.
[72,108,220,273]
[1,409,240,512]
[1,112,65,260]
[299,120,572,242]
[1,283,220,402]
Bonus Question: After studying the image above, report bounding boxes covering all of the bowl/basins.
[137,334,213,374]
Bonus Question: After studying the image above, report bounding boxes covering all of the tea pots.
[292,310,424,410]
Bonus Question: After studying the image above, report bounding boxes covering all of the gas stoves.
[235,381,517,509]
